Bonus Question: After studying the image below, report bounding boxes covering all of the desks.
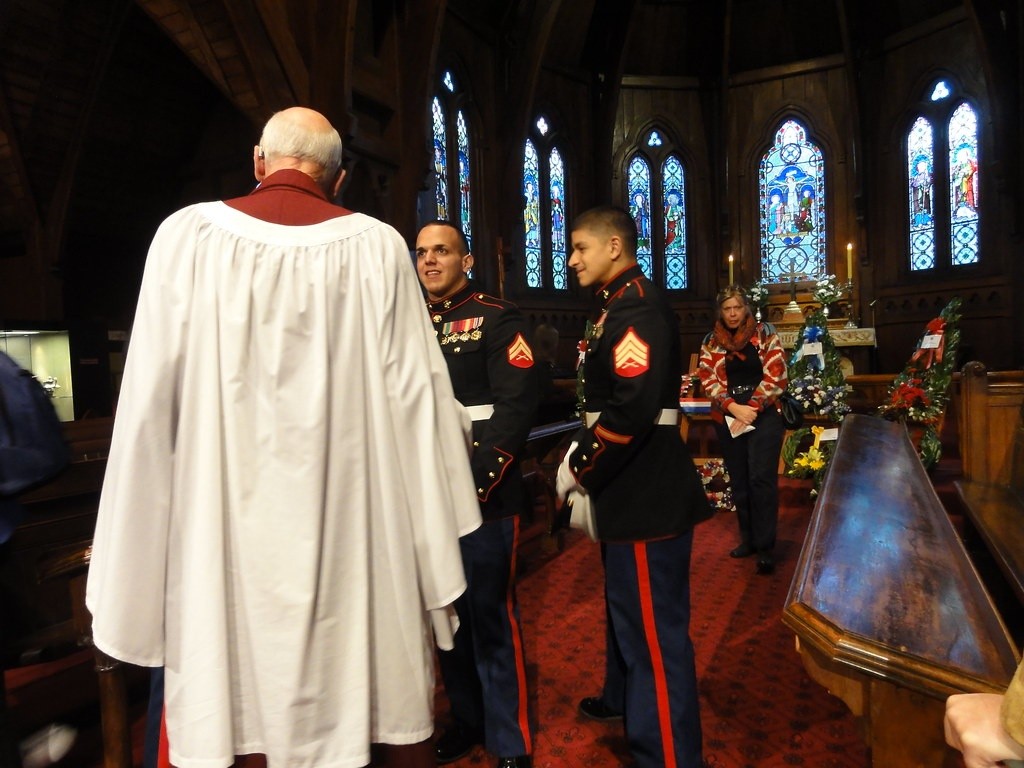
[777,327,877,380]
[844,372,960,441]
[783,411,1023,767]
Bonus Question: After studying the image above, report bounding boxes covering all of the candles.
[729,261,733,285]
[847,248,852,279]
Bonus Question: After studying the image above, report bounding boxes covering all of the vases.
[755,308,762,324]
[822,304,830,317]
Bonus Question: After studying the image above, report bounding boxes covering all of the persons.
[530,323,582,428]
[415,221,540,768]
[85,103,482,768]
[944,656,1024,768]
[555,205,718,768]
[700,285,787,578]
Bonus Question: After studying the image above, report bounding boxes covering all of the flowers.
[788,370,935,473]
[926,317,947,335]
[746,280,768,306]
[814,273,843,304]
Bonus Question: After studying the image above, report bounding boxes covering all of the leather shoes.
[757,553,777,574]
[731,543,756,558]
[498,754,532,768]
[433,724,485,762]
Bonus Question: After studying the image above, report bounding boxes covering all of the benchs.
[954,360,1024,609]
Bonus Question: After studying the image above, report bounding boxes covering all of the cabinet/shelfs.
[0,380,582,671]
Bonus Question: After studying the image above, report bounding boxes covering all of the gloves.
[556,440,577,502]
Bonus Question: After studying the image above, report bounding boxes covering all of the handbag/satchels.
[779,390,804,431]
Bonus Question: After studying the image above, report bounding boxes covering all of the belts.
[464,404,495,421]
[581,407,678,430]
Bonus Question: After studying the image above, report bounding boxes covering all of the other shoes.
[579,696,624,720]
